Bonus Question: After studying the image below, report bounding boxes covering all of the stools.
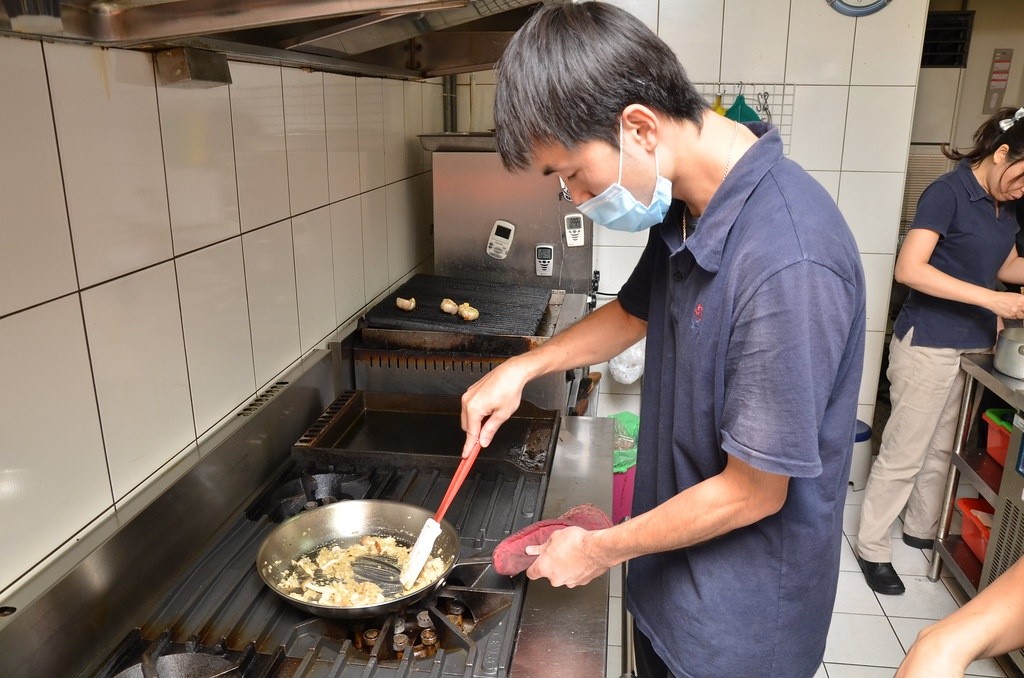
[849,419,873,491]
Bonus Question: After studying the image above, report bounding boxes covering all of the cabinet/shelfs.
[928,353,1024,674]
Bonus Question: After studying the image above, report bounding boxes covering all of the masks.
[575,118,672,232]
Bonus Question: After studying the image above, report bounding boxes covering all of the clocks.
[826,0,891,17]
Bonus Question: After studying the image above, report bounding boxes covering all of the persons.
[461,1,867,678]
[894,553,1024,678]
[856,106,1024,595]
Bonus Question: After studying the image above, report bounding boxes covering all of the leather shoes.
[858,554,905,594]
[902,532,935,549]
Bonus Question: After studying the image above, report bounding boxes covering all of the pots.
[993,328,1024,380]
[256,499,630,615]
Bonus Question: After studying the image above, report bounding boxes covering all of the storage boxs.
[983,409,1017,468]
[957,498,995,566]
